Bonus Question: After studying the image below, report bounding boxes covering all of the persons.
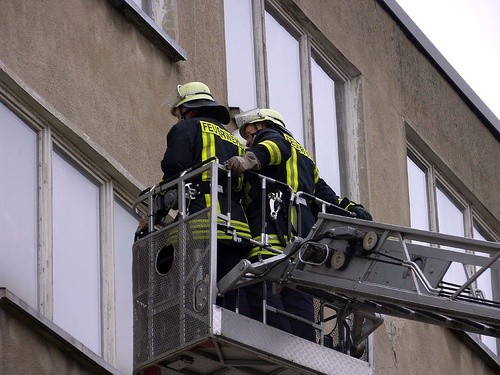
[311,193,372,221]
[227,105,337,342]
[136,79,256,311]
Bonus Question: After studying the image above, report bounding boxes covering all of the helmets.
[160,81,215,114]
[234,108,285,139]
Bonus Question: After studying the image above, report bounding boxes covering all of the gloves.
[223,151,261,174]
[134,210,149,242]
[350,203,373,221]
